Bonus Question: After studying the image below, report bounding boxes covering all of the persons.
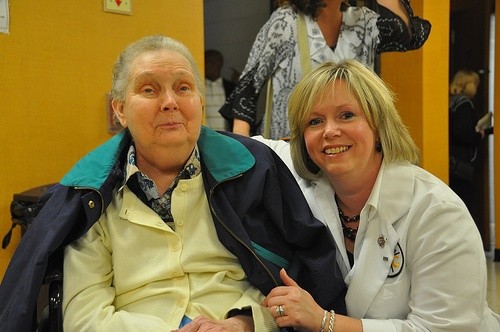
[218,0,432,142]
[249,58,500,332]
[0,35,348,332]
[203,49,241,132]
[449,70,492,203]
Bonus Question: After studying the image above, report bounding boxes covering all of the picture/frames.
[103,0,132,15]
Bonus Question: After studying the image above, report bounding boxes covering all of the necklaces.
[338,208,360,240]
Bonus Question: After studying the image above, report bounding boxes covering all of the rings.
[276,305,283,317]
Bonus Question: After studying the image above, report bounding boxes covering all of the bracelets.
[328,310,335,332]
[321,310,327,332]
